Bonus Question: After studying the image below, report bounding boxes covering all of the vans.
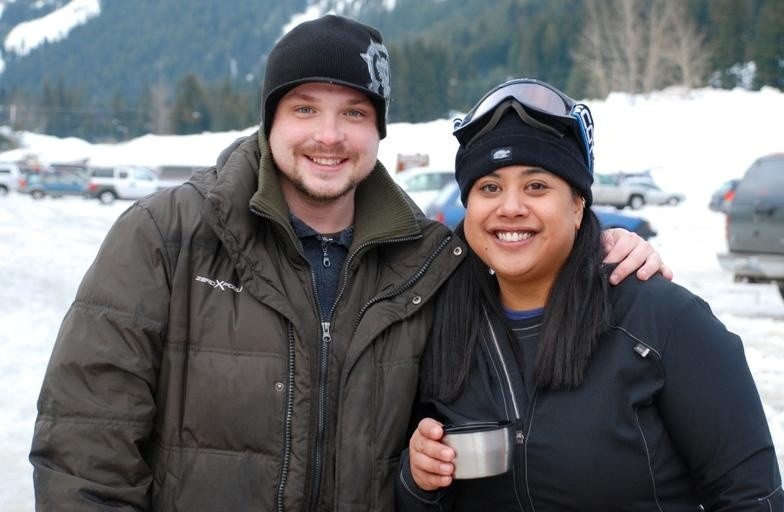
[708,150,784,296]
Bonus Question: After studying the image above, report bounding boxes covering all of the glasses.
[453,78,594,174]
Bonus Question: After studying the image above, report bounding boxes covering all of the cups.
[433,421,516,479]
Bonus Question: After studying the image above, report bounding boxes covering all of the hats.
[261,15,390,140]
[454,112,593,209]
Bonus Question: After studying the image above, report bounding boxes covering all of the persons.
[30,16,674,512]
[396,80,783,512]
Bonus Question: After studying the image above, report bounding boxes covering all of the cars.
[397,163,684,238]
[0,159,213,205]
